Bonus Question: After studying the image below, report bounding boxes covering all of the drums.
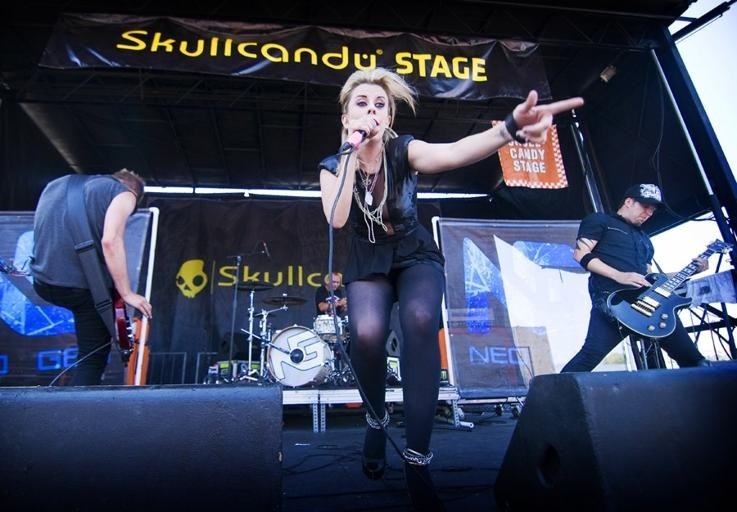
[267,325,332,388]
[322,318,350,343]
[313,315,340,335]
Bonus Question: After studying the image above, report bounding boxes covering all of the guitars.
[113,291,133,352]
[607,238,733,339]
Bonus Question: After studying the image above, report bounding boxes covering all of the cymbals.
[263,296,307,306]
[234,282,274,291]
[249,313,276,318]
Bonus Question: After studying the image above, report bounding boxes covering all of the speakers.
[384,329,400,357]
[494,363,736,511]
[0,383,282,512]
[220,331,260,360]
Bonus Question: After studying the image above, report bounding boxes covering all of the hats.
[623,182,667,213]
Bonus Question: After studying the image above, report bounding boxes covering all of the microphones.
[260,240,271,262]
[342,120,378,151]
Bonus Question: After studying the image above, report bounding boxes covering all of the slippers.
[403,461,442,512]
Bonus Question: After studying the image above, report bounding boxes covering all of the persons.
[32,165,153,387]
[560,183,708,371]
[314,272,347,315]
[318,67,589,511]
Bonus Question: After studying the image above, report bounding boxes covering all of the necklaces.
[340,141,392,205]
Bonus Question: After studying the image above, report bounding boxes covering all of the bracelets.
[503,111,529,147]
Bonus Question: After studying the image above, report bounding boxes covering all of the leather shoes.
[363,423,387,478]
